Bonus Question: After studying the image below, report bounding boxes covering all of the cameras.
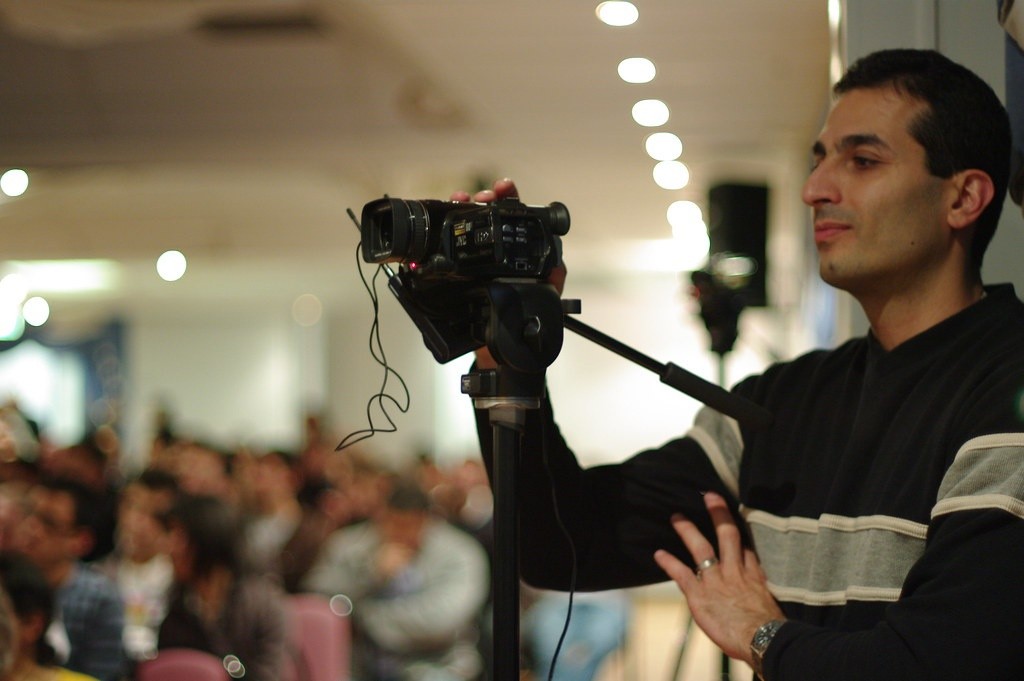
[361,196,570,285]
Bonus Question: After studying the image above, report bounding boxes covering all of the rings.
[699,558,716,570]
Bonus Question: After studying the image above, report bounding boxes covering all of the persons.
[447,47,1024,681]
[0,400,633,681]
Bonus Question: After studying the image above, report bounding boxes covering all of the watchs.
[748,619,785,681]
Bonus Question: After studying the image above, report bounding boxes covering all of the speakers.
[708,182,770,306]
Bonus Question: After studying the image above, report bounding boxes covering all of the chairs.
[283,594,351,681]
[134,648,230,681]
